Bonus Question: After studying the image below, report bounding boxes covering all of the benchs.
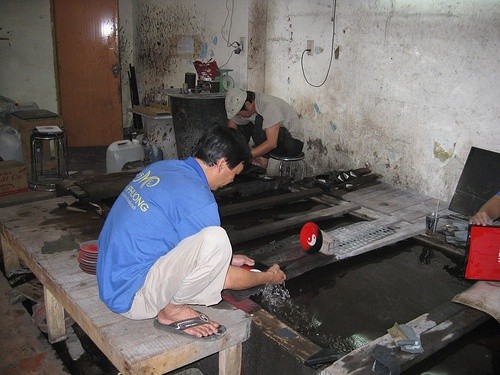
[0,195,252,375]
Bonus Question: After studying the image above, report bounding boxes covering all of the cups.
[426,212,440,235]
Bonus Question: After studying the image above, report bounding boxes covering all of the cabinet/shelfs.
[5,107,65,175]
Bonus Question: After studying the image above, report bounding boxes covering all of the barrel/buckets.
[106,140,144,174]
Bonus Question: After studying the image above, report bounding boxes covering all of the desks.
[134,111,180,160]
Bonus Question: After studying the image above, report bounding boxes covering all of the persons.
[96,125,285,342]
[469,191,500,226]
[225,87,304,167]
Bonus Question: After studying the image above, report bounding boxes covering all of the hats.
[224,87,247,120]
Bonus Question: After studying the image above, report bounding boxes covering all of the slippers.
[152,311,226,342]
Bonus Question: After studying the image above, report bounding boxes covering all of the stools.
[28,127,68,191]
[268,151,307,179]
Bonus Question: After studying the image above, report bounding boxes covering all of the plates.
[239,262,268,273]
[300,222,323,254]
[78,241,97,275]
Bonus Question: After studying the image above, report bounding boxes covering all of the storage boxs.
[0,159,28,196]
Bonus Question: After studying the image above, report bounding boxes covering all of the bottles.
[143,86,176,107]
